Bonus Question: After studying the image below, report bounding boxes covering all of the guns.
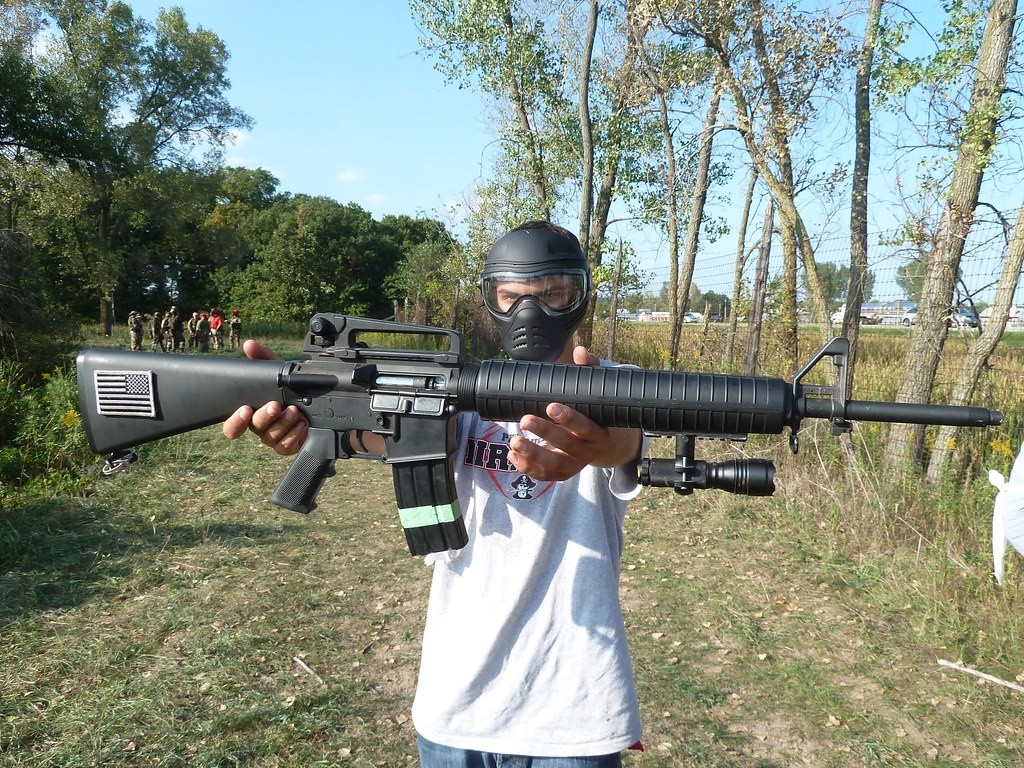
[78,313,1002,557]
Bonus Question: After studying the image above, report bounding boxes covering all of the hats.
[200,314,207,320]
[193,312,198,315]
[232,309,240,313]
[129,311,138,316]
[210,308,217,315]
[136,312,141,317]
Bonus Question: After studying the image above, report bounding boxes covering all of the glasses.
[155,315,160,317]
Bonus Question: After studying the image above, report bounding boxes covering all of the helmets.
[475,227,592,362]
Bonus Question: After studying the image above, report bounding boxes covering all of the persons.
[127,306,241,355]
[222,221,651,768]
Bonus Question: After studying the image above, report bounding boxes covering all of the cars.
[683,312,698,324]
[859,316,875,325]
[902,306,979,328]
[709,315,723,324]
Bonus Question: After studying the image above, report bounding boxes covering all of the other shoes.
[131,343,241,354]
